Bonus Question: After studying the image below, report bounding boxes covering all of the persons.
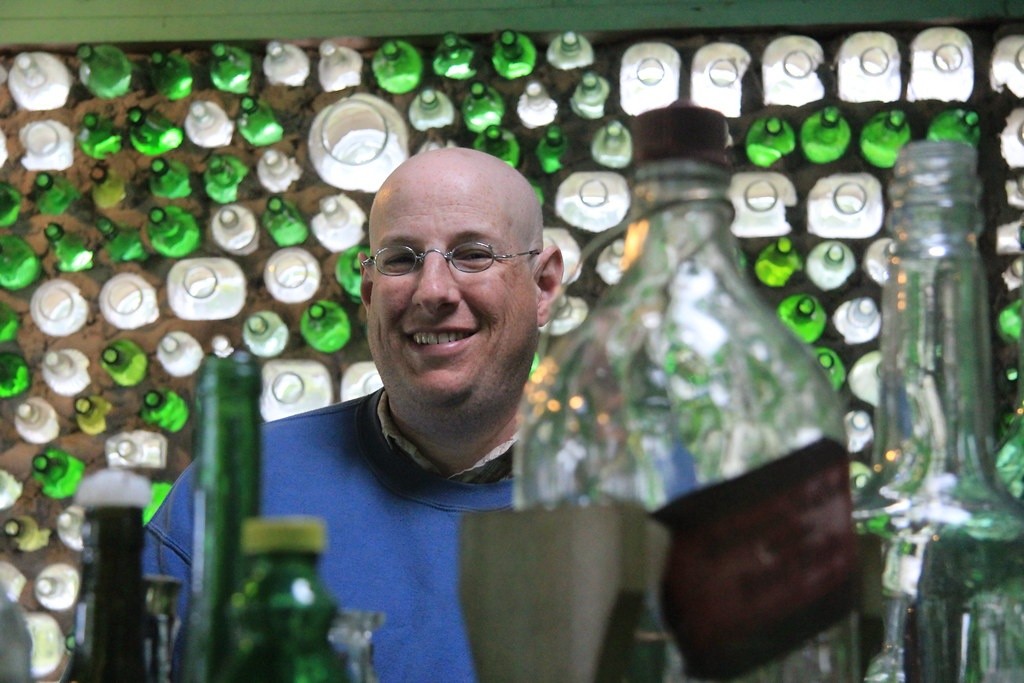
[144,146,697,682]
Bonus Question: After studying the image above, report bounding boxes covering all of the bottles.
[328,609,378,682]
[854,143,1023,683]
[515,105,859,682]
[59,468,181,683]
[204,514,337,682]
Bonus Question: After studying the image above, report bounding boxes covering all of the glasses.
[362,240,542,277]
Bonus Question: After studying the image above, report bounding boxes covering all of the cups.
[904,522,1024,683]
[459,508,620,681]
